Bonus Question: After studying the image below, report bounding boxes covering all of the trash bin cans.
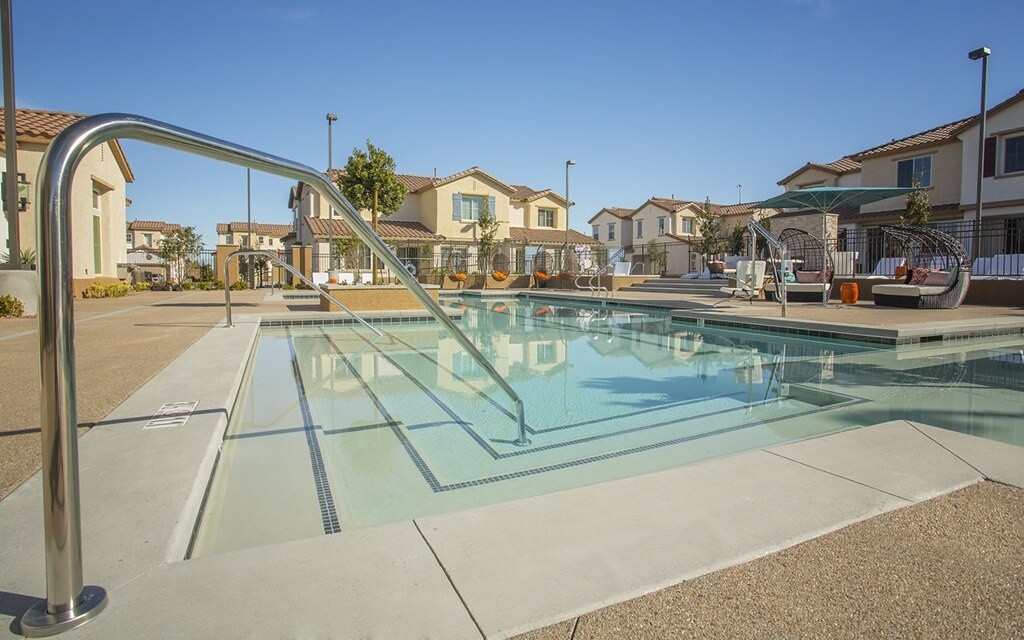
[328,271,339,283]
[841,283,858,304]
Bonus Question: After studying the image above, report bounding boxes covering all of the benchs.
[766,282,831,302]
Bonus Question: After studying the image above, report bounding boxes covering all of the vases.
[841,282,859,304]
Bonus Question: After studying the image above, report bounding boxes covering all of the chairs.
[720,275,773,300]
[872,271,951,297]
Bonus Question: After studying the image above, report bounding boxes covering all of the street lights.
[326,113,338,270]
[566,160,576,250]
[969,47,991,260]
